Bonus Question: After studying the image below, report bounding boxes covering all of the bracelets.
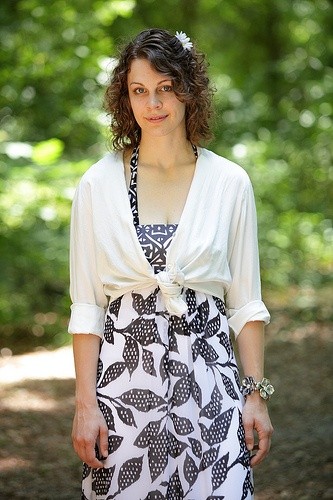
[240,376,275,401]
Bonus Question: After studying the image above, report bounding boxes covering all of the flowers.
[174,29,194,51]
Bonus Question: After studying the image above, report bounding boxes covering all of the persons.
[67,29,274,500]
